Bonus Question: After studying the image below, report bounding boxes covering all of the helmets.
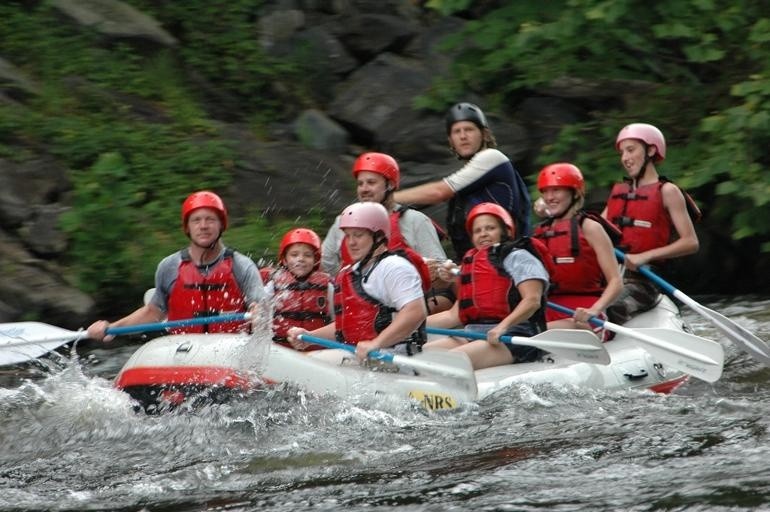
[615,122,667,160]
[536,162,585,190]
[352,151,400,189]
[279,228,322,270]
[465,201,516,241]
[339,201,391,243]
[181,191,229,233]
[444,102,488,136]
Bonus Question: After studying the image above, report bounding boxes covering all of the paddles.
[542,299,725,384]
[419,327,612,367]
[616,248,769,367]
[294,333,478,399]
[0,311,257,367]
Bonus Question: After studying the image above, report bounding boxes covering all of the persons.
[265,228,335,353]
[423,203,550,373]
[534,122,699,328]
[394,102,531,266]
[87,191,267,343]
[317,151,449,282]
[531,162,624,344]
[286,201,428,371]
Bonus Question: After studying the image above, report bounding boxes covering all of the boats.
[113,294,688,415]
